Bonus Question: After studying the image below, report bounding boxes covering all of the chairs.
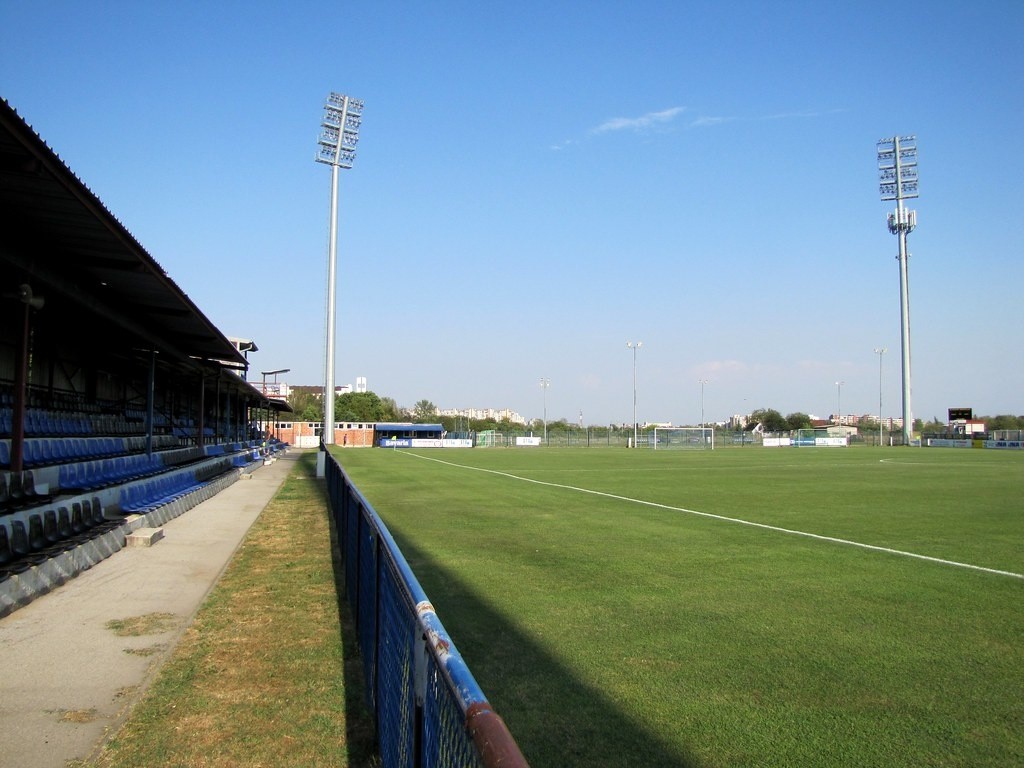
[0,399,286,582]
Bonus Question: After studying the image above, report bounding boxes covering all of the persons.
[265,426,270,457]
[249,418,257,440]
[344,434,347,446]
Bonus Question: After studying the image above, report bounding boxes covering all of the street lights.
[319,89,366,449]
[878,134,920,446]
